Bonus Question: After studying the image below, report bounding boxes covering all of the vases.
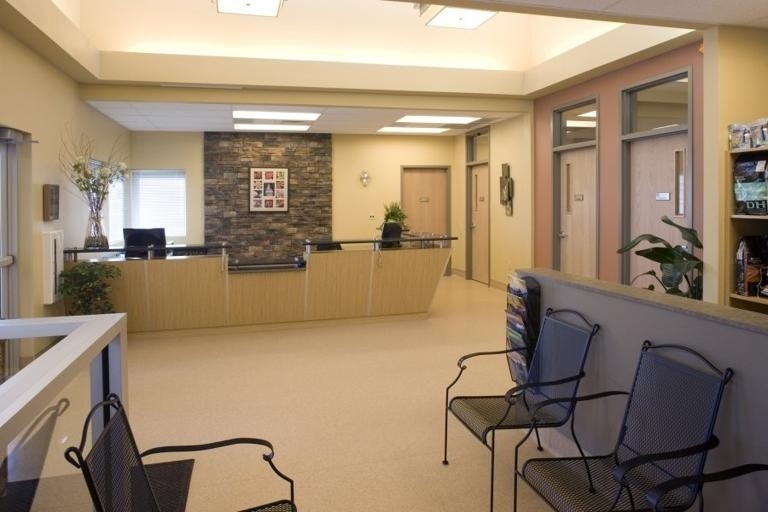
[84,210,109,248]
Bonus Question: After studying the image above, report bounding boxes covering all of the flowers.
[58,122,131,210]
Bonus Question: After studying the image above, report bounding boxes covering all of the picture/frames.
[249,167,289,212]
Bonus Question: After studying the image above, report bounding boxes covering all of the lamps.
[216,0,500,30]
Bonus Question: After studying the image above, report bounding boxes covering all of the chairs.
[316,243,342,250]
[173,248,207,255]
[0,398,70,512]
[65,392,297,512]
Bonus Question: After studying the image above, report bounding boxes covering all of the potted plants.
[377,201,409,247]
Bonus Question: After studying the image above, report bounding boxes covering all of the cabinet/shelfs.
[724,145,767,315]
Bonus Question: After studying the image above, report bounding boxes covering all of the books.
[503,273,538,392]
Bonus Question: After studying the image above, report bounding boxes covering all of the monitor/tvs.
[381,222,402,248]
[123,228,167,258]
